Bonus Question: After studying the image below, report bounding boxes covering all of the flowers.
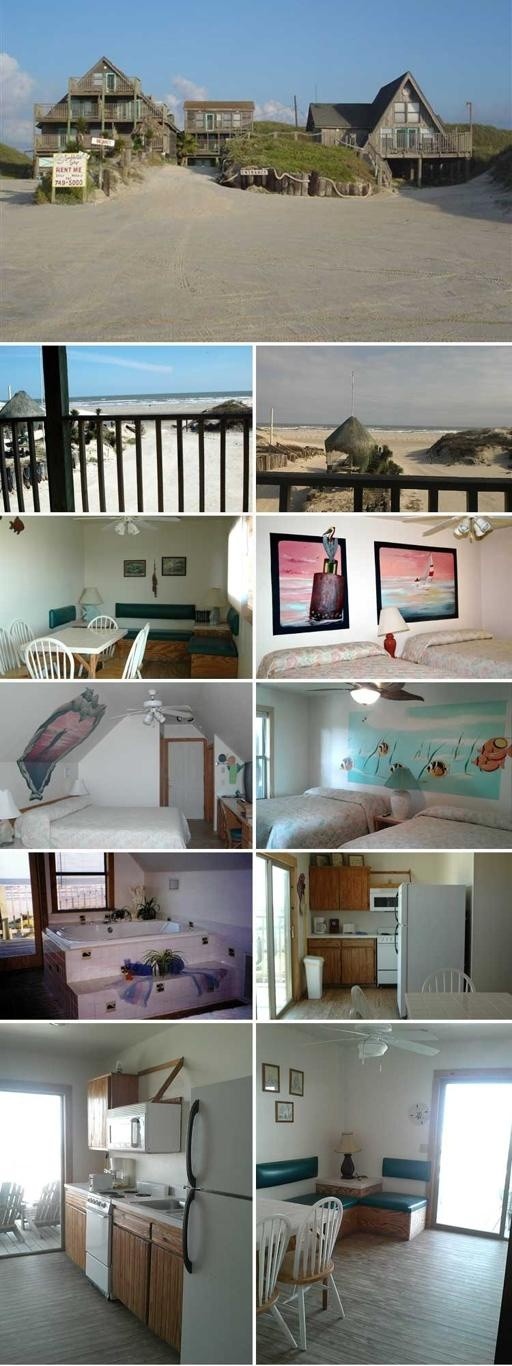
[128,886,159,920]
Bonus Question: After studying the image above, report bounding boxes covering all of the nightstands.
[369,810,415,832]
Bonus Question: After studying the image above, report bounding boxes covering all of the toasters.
[344,923,355,933]
[88,1174,114,1192]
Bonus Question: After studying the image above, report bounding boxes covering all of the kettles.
[330,919,340,933]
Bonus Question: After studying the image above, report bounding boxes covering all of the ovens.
[84,1207,112,1267]
[377,944,397,971]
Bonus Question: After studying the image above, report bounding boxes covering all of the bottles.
[80,916,85,924]
[104,913,110,922]
[235,790,240,796]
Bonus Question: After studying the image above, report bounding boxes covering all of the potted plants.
[140,944,183,977]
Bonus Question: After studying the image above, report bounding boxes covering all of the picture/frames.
[261,1060,282,1094]
[372,538,461,628]
[273,1100,294,1124]
[122,558,147,579]
[160,556,188,577]
[288,1067,306,1098]
[312,853,366,868]
[268,531,351,638]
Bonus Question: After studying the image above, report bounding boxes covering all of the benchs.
[111,601,197,660]
[256,1157,358,1238]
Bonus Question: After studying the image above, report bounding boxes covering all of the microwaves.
[106,1103,181,1153]
[369,888,399,913]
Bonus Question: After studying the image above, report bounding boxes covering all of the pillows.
[20,794,97,824]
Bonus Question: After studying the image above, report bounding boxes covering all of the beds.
[10,794,193,850]
[257,784,390,850]
[398,629,512,679]
[338,804,512,850]
[254,637,464,682]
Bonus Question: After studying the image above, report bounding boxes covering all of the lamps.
[78,585,104,621]
[384,766,421,821]
[0,788,23,845]
[452,517,493,541]
[142,689,167,727]
[333,1130,363,1180]
[113,517,143,537]
[67,779,90,799]
[199,586,233,627]
[348,682,386,714]
[374,604,411,658]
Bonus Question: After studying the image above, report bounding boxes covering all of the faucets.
[183,1185,191,1189]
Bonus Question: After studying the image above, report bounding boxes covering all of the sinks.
[128,1198,186,1211]
[157,1211,185,1222]
[46,919,210,951]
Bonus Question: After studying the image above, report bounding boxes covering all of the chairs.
[416,966,482,994]
[31,1177,65,1240]
[1,1182,28,1247]
[49,606,76,630]
[187,608,239,678]
[217,795,248,849]
[0,614,151,679]
[356,1158,433,1244]
[279,1196,349,1354]
[347,982,399,1020]
[256,1213,300,1354]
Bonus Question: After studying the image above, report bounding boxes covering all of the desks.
[255,1190,341,1251]
[215,793,253,849]
[191,623,232,641]
[317,1178,382,1197]
[403,988,512,1021]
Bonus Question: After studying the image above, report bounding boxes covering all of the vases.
[143,910,156,919]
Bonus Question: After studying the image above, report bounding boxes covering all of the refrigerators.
[181,1075,253,1363]
[394,883,467,1019]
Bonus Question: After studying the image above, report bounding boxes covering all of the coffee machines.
[313,917,328,934]
[106,1158,128,1187]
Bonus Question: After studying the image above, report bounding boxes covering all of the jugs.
[380,932,398,937]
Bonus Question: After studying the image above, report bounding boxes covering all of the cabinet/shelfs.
[59,1182,90,1269]
[109,1191,186,1354]
[306,864,372,913]
[306,933,378,987]
[85,1071,140,1156]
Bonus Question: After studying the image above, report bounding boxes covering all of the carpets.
[182,1005,251,1020]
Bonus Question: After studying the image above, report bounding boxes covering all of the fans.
[305,683,433,709]
[299,1023,444,1068]
[107,687,195,726]
[400,516,511,542]
[70,515,185,538]
